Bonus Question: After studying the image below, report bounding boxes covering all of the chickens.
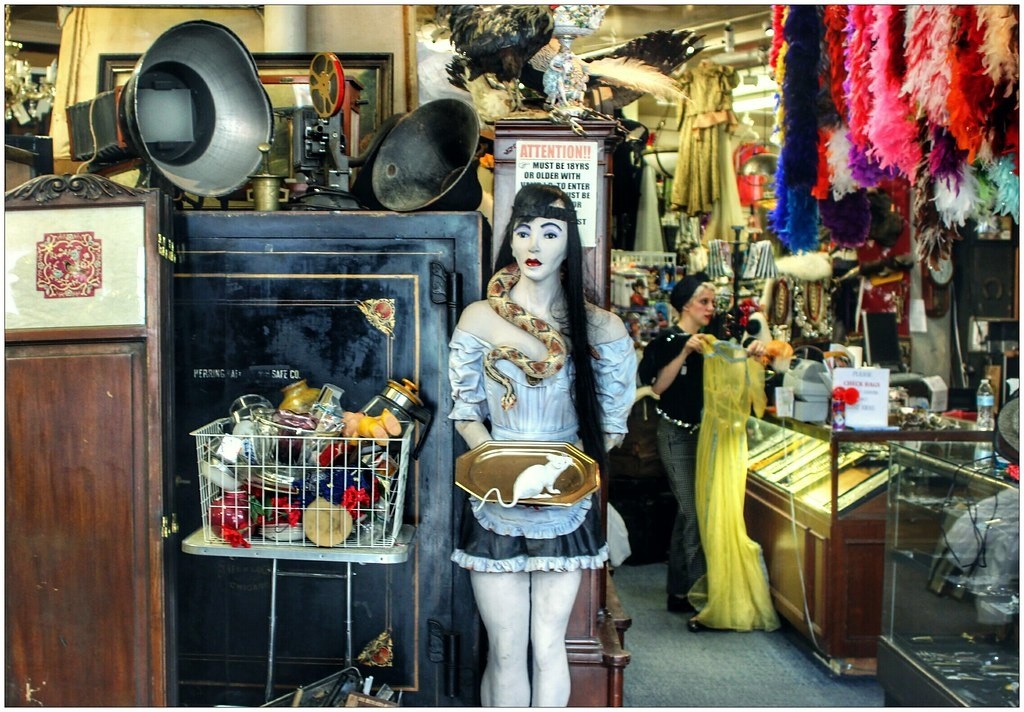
[437,4,562,112]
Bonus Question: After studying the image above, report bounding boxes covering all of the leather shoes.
[667,591,706,613]
[689,615,735,632]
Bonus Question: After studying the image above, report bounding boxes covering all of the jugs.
[355,378,434,460]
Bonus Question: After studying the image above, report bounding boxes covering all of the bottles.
[974,442,992,473]
[278,380,322,414]
[976,379,995,432]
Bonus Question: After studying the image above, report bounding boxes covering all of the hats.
[669,271,711,313]
[998,398,1020,452]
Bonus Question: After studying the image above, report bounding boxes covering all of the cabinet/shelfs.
[869,441,1020,707]
[4,174,166,708]
[161,211,490,708]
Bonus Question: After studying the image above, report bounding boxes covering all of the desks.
[181,528,418,698]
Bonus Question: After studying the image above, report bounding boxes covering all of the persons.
[447,181,637,708]
[629,277,662,309]
[543,45,572,106]
[637,272,768,615]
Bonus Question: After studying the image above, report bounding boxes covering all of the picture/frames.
[98,50,395,210]
